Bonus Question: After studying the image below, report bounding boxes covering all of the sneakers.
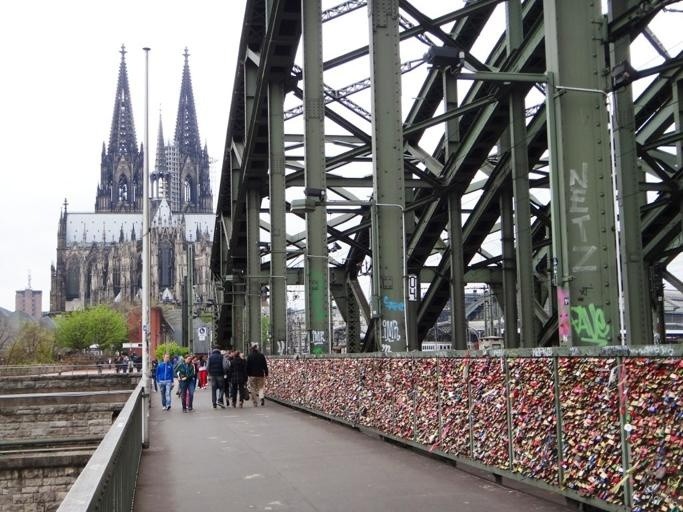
[183,407,192,413]
[162,405,170,410]
[214,398,265,410]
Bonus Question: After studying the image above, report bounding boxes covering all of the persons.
[97,341,270,413]
